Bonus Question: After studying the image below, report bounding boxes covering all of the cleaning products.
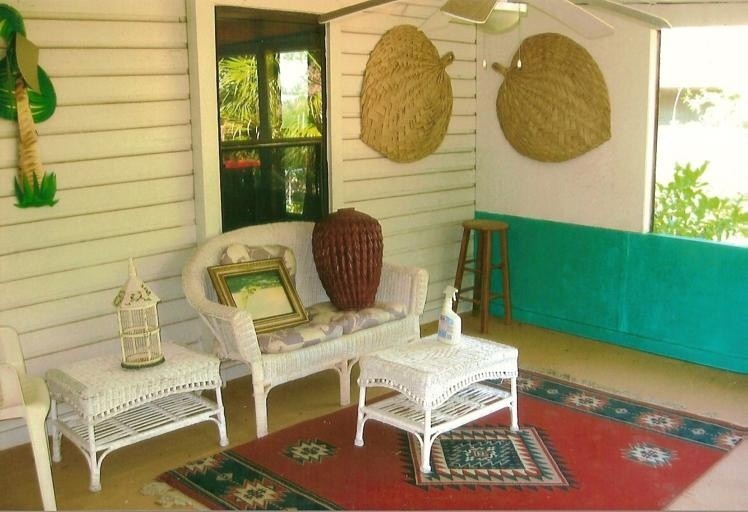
[438,285,463,345]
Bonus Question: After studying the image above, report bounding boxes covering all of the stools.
[453,219,510,334]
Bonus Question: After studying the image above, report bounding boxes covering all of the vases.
[311,208,384,310]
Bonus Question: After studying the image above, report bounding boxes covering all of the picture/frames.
[207,257,310,332]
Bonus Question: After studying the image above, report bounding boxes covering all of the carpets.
[139,363,748,511]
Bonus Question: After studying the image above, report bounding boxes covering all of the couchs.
[180,221,431,439]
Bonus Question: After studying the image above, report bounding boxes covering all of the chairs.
[1,325,58,511]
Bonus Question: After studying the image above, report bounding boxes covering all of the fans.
[311,0,670,37]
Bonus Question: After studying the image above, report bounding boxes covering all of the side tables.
[45,343,229,494]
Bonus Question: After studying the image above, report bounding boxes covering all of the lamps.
[482,2,528,35]
